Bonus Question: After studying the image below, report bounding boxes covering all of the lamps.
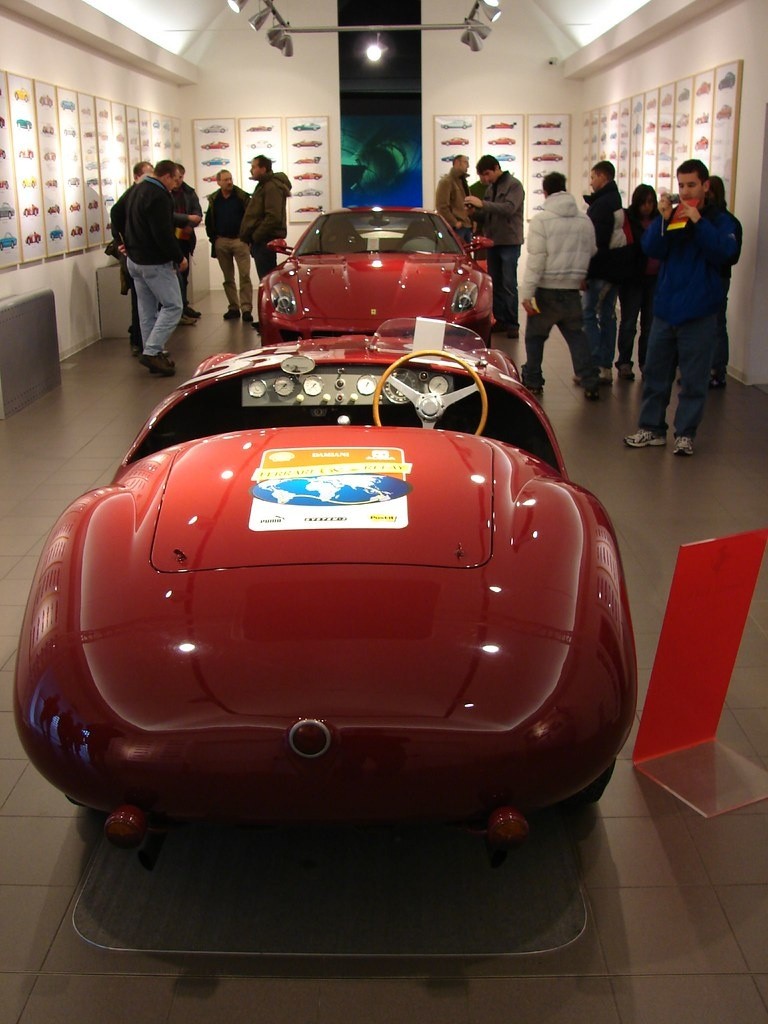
[468,19,492,39]
[228,0,250,14]
[461,30,468,45]
[269,36,285,50]
[467,31,484,52]
[478,1,502,24]
[247,6,271,32]
[484,0,500,7]
[268,24,283,41]
[367,32,390,62]
[281,35,294,57]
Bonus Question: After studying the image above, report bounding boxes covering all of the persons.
[238,154,292,329]
[465,155,526,338]
[571,160,627,385]
[520,172,602,396]
[104,160,188,374]
[173,164,203,327]
[204,170,256,323]
[613,183,664,380]
[435,155,474,243]
[623,159,743,458]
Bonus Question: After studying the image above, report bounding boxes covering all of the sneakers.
[622,429,667,447]
[672,436,692,455]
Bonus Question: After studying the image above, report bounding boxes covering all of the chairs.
[398,220,448,254]
[317,218,367,252]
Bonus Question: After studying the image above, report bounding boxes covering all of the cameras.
[465,203,473,208]
[668,194,680,204]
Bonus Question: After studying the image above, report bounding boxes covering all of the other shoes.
[598,366,613,382]
[179,314,197,325]
[138,351,177,376]
[130,343,144,356]
[182,305,202,317]
[241,311,253,321]
[249,321,261,334]
[676,369,725,391]
[223,310,241,319]
[522,376,543,394]
[618,363,636,380]
[585,388,600,402]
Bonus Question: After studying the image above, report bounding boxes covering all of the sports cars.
[257,206,496,351]
[12,316,639,871]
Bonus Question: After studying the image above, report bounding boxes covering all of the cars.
[292,121,321,131]
[246,125,273,132]
[441,136,469,146]
[201,156,231,166]
[200,123,227,133]
[441,153,470,163]
[533,121,562,129]
[581,71,737,205]
[535,137,562,146]
[493,153,516,162]
[200,140,230,149]
[440,119,472,129]
[532,152,563,162]
[532,169,557,178]
[203,173,217,181]
[248,139,324,213]
[486,122,516,130]
[0,86,183,250]
[488,137,516,145]
